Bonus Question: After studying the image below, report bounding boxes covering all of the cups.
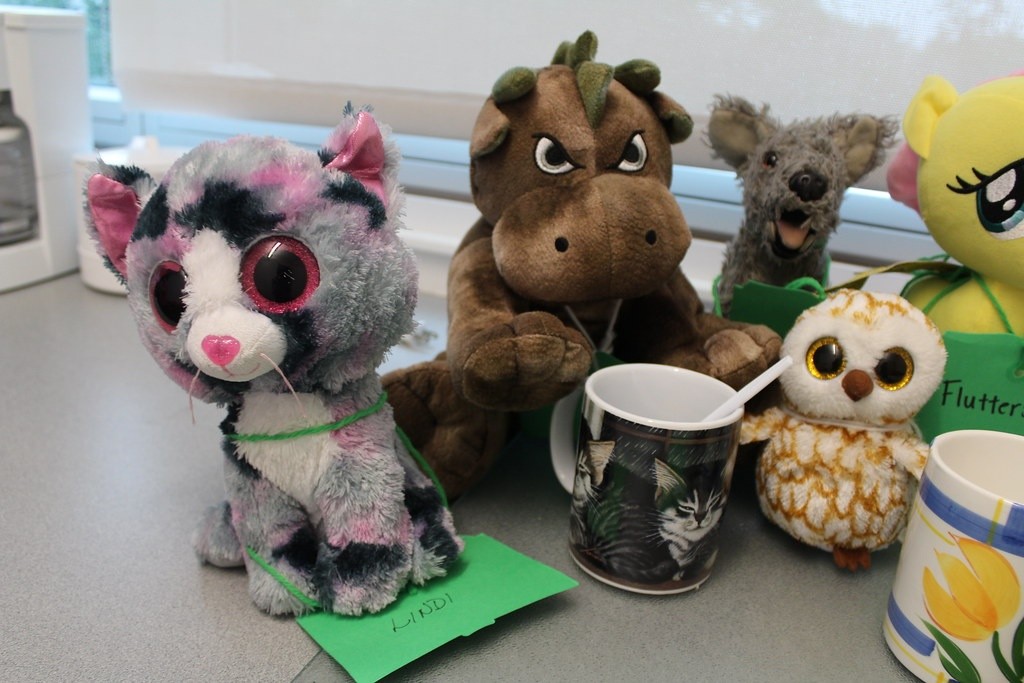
[882,429,1024,683]
[75,134,191,296]
[550,363,745,595]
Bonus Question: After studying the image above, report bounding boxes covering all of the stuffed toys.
[739,289,947,572]
[83,101,464,617]
[887,69,1024,444]
[708,94,899,322]
[379,30,783,503]
